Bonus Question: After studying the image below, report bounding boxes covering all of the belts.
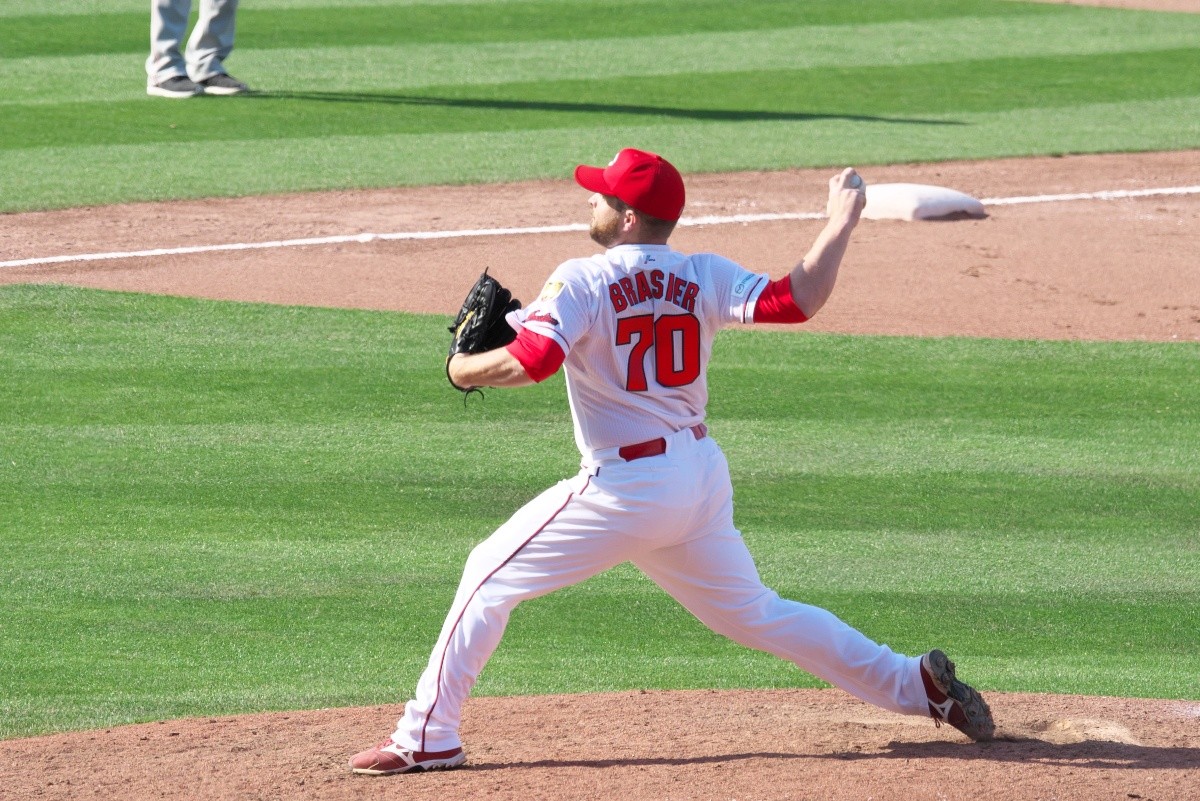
[617,423,709,462]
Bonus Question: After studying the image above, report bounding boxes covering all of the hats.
[576,148,685,221]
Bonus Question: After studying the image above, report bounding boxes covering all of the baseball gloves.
[444,266,525,409]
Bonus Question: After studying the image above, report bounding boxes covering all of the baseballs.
[850,173,862,188]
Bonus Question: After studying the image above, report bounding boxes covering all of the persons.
[146,0,248,97]
[348,146,995,774]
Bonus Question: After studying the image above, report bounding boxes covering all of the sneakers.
[919,649,995,744]
[349,736,467,775]
[196,73,247,95]
[147,76,202,98]
